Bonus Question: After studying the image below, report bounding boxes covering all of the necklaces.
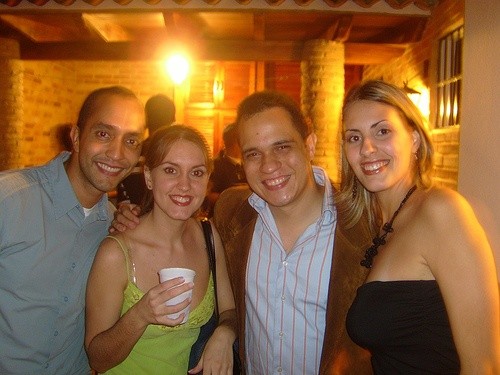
[359,183,419,269]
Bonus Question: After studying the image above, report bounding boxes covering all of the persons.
[209,124,247,194]
[342,82,495,374]
[109,92,372,374]
[0,87,145,375]
[118,94,175,212]
[86,127,238,375]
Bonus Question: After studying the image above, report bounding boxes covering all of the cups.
[157,267,196,325]
